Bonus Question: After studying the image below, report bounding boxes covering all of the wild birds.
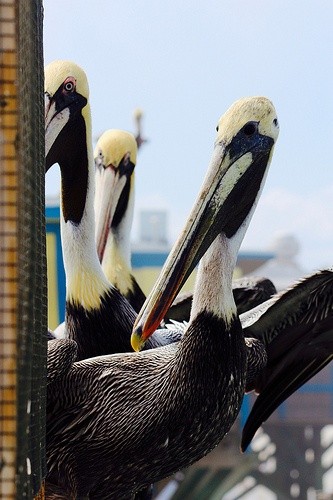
[44,60,333,500]
